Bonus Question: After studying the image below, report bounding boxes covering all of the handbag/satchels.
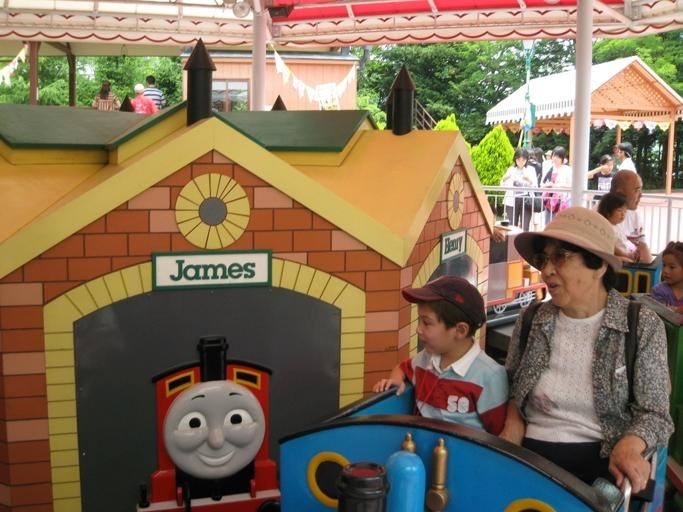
[523,185,570,215]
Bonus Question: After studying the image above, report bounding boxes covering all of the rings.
[641,479,647,483]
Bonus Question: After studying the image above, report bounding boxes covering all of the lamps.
[233,1,253,17]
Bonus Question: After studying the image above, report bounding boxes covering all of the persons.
[92,79,120,111]
[498,143,573,232]
[650,240,683,313]
[596,192,650,264]
[616,141,637,171]
[371,273,508,435]
[143,75,165,111]
[588,153,617,193]
[608,144,621,173]
[609,168,643,238]
[131,84,159,114]
[497,206,675,496]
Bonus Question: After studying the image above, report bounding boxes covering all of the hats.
[133,83,145,94]
[512,205,626,275]
[400,275,488,329]
